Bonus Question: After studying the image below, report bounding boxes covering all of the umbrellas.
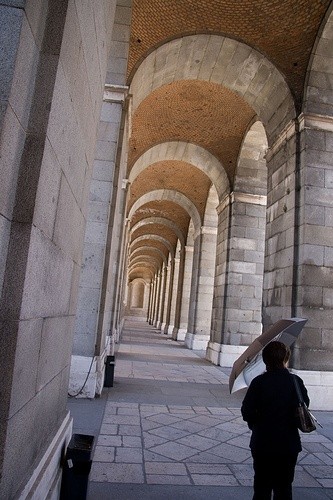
[229,317,325,429]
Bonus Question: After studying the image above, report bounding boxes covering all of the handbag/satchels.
[293,374,317,433]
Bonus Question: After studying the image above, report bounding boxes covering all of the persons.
[241,341,317,500]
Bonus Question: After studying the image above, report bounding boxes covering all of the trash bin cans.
[105,355,115,387]
[59,433,97,500]
